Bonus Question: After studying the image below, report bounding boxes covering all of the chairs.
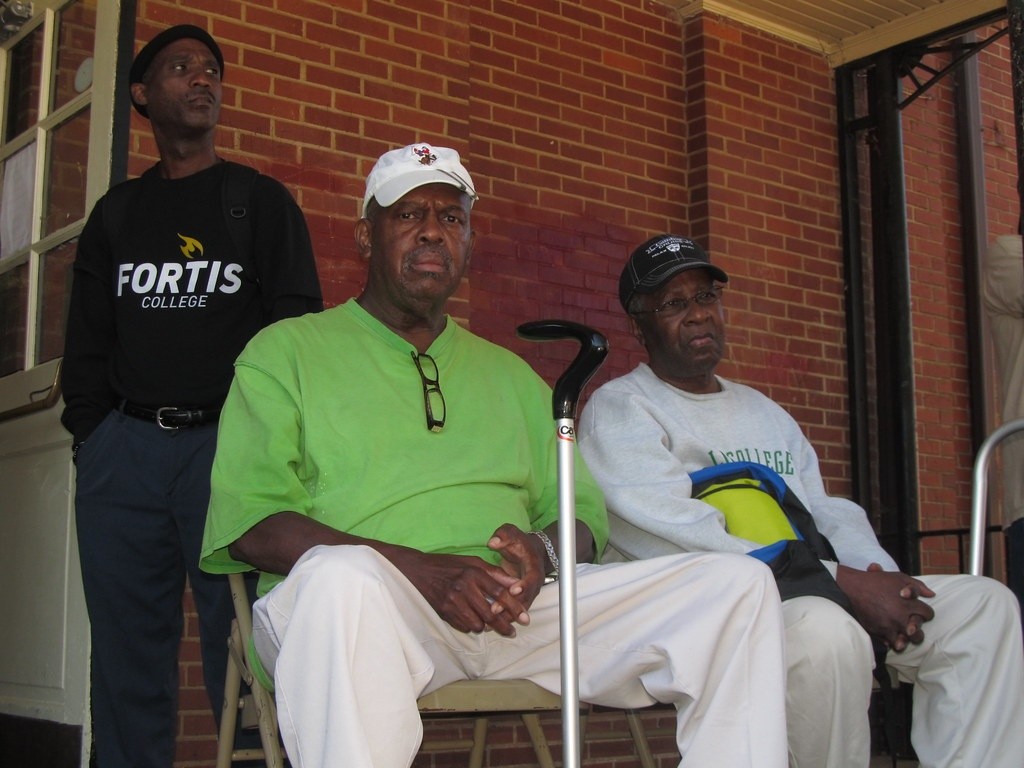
[215,573,660,767]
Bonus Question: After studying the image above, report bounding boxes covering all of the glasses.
[631,285,723,314]
[411,351,446,433]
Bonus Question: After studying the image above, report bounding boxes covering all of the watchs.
[527,529,560,585]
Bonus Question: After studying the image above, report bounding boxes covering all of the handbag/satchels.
[688,462,854,615]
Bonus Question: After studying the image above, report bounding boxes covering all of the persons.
[60,26,323,767]
[575,232,1024,768]
[197,142,785,767]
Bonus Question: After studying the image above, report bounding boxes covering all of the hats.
[618,234,729,314]
[128,24,224,118]
[362,142,479,219]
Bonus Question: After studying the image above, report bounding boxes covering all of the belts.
[115,399,222,430]
